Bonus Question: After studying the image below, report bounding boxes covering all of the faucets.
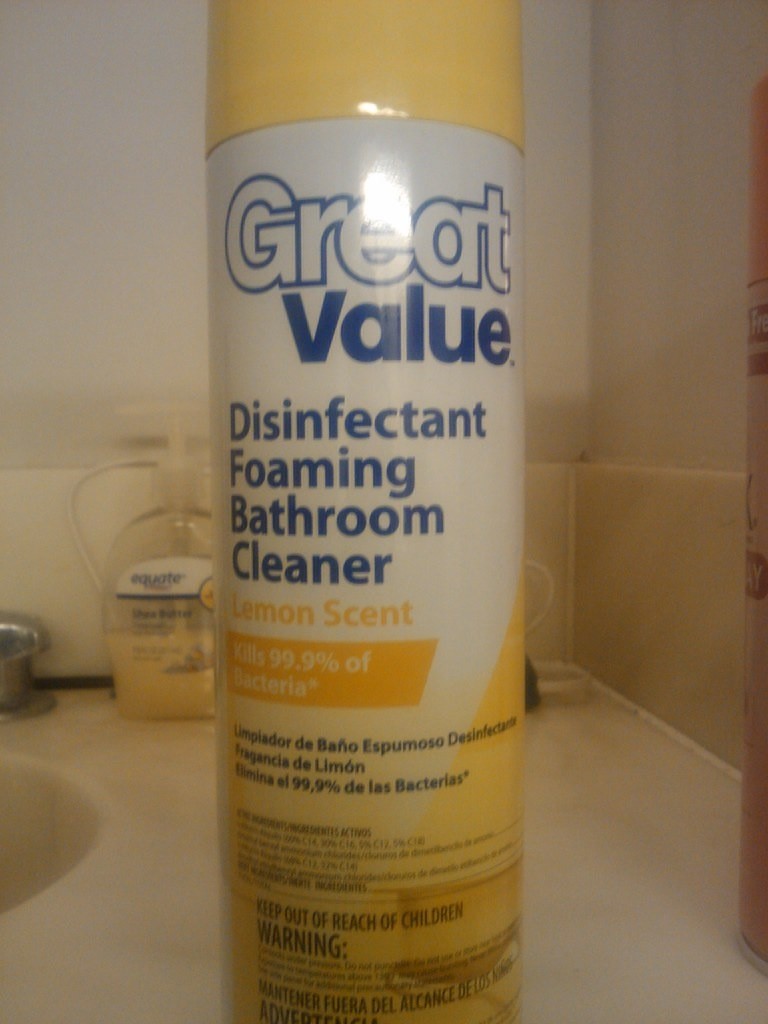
[0,612,55,718]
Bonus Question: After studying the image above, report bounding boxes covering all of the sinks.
[0,749,111,915]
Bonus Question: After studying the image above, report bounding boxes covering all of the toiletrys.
[103,412,215,722]
